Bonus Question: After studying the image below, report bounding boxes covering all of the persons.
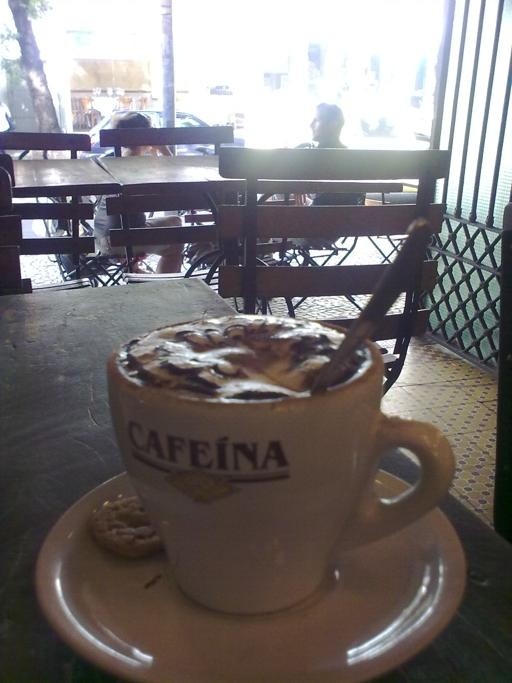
[92,110,184,274]
[268,101,366,251]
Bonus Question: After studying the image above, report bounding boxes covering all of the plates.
[34,456,467,681]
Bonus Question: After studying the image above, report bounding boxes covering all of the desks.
[0,274,511,681]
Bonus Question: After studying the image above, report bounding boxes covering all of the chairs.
[215,146,452,394]
[102,182,242,300]
[0,123,91,160]
[1,167,36,296]
[97,125,234,156]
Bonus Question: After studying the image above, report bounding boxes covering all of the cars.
[76,109,248,162]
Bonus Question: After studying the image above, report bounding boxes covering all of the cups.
[108,316,456,614]
